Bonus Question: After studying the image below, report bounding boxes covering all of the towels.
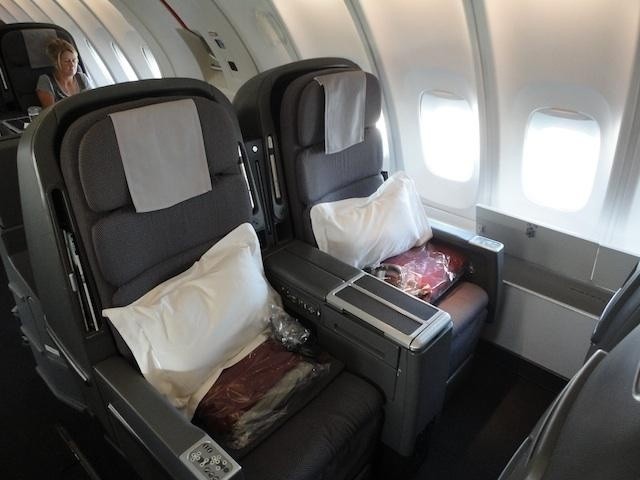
[311,69,367,156]
[106,97,213,214]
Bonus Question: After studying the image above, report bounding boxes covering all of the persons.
[36,33,91,110]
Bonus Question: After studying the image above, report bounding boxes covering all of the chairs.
[2,76,455,480]
[496,256,640,479]
[1,21,90,230]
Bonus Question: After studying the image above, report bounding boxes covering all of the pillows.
[102,221,284,423]
[309,169,434,272]
